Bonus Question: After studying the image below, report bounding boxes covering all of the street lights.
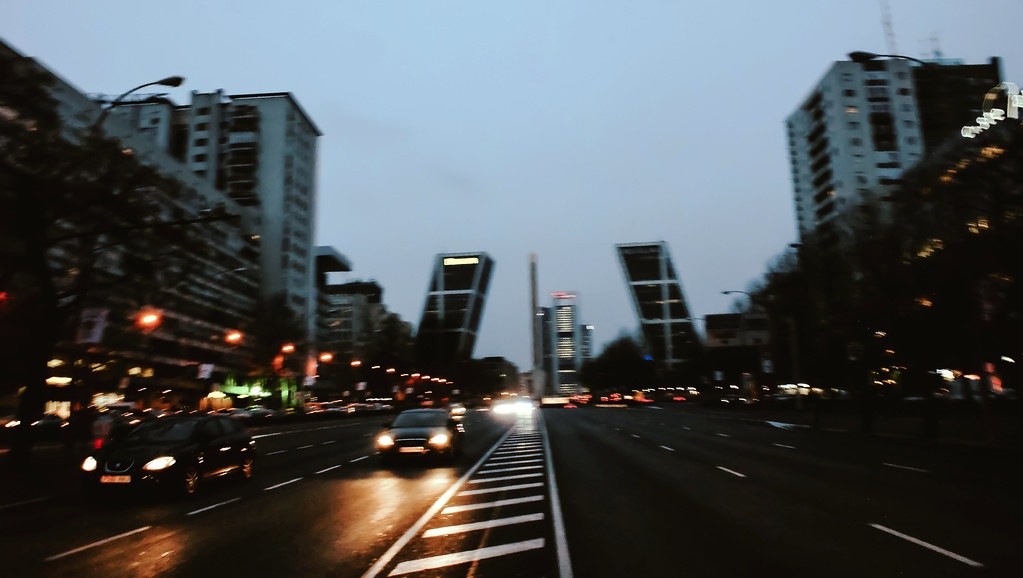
[83,77,185,292]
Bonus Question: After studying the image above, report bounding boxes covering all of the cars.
[208,398,393,420]
[376,408,460,466]
[80,414,257,504]
